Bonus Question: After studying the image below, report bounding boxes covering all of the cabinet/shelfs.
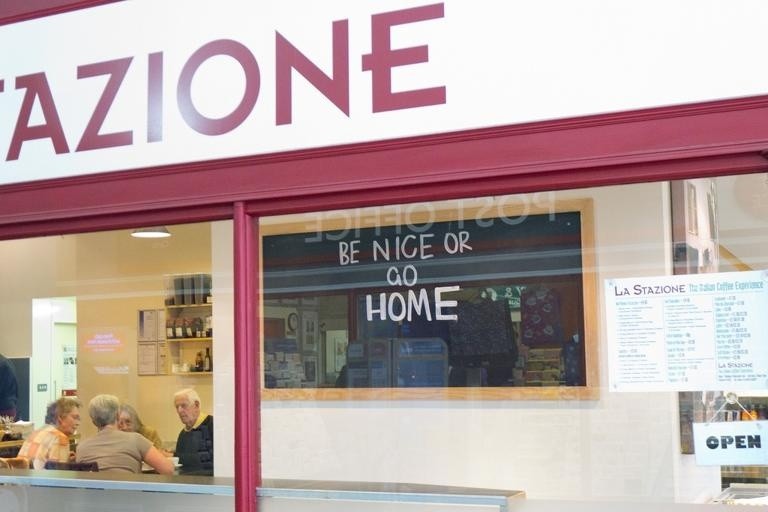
[164,302,213,375]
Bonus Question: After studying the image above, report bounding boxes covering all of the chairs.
[0,457,99,472]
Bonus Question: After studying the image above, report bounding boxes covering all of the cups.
[168,457,179,464]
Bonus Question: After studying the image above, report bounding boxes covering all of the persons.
[15,397,82,470]
[118,403,166,452]
[163,388,214,476]
[75,393,176,478]
[0,352,21,425]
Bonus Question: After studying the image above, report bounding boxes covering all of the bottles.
[726,403,768,422]
[162,273,212,307]
[196,347,212,371]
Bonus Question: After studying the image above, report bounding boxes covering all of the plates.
[139,461,183,472]
